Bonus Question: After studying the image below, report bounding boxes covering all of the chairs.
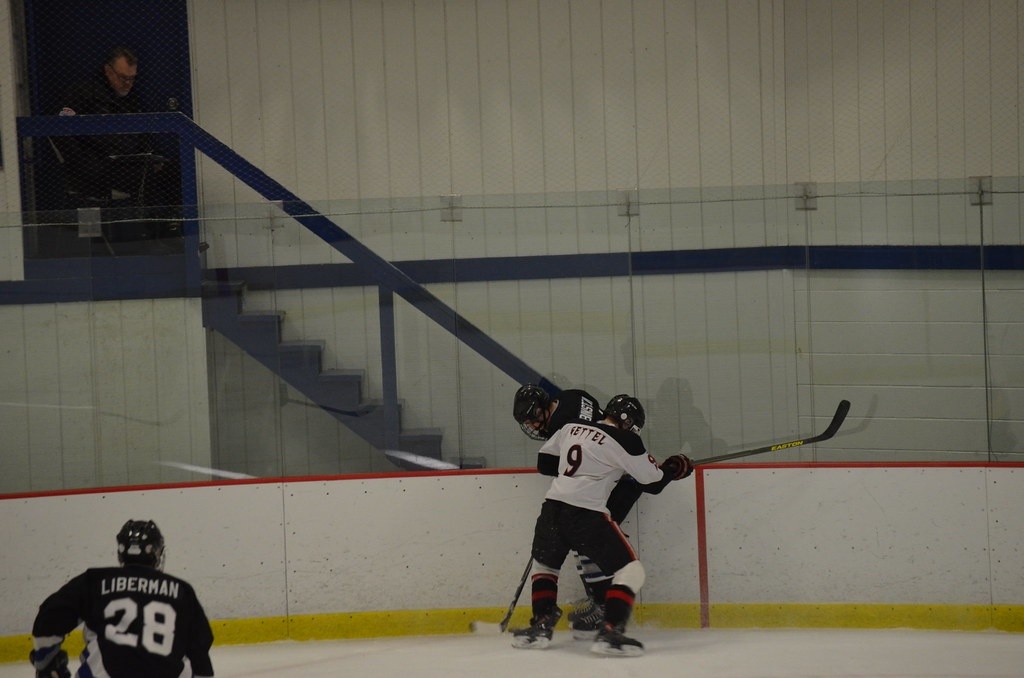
[40,110,172,260]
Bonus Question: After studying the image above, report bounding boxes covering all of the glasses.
[108,66,136,82]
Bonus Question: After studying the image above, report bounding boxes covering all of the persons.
[514,394,694,649]
[513,383,645,631]
[58,48,180,241]
[30,520,215,678]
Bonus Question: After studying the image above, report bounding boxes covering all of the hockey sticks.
[622,399,851,482]
[469,555,534,634]
[47,135,117,259]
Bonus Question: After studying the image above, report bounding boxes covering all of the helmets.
[513,383,551,441]
[116,519,166,571]
[605,394,645,436]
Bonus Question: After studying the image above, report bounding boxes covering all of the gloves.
[664,454,694,480]
[29,642,71,678]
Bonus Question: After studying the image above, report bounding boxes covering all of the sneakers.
[591,620,645,656]
[511,606,563,648]
[568,599,596,629]
[568,604,606,640]
